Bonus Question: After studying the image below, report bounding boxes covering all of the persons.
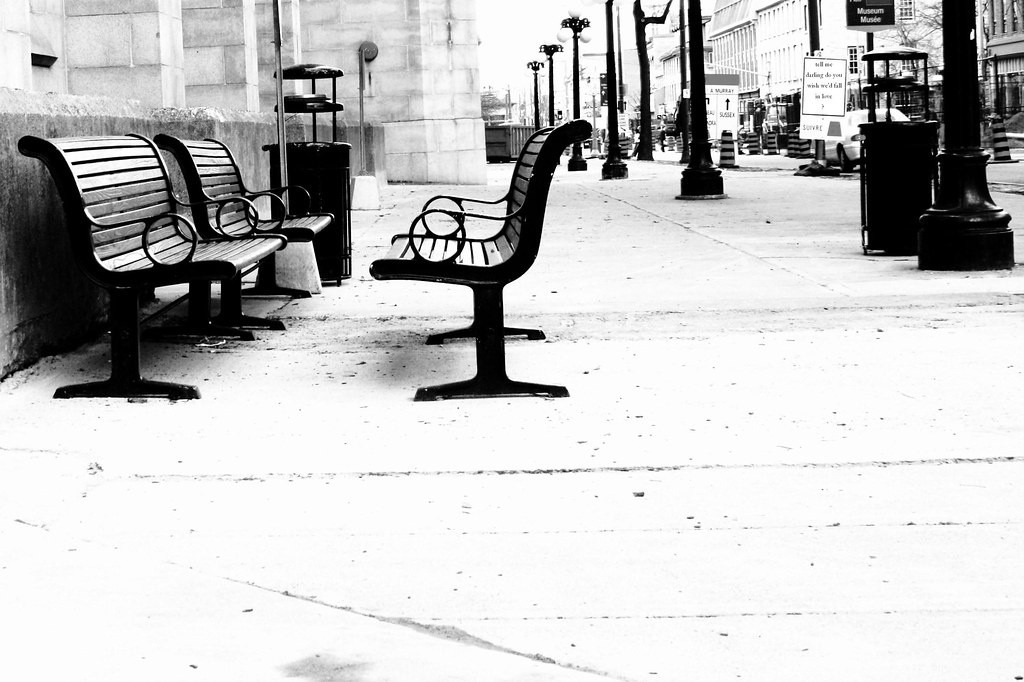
[658,128,667,153]
[632,128,640,154]
[753,107,765,140]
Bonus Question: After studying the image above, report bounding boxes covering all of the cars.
[821,108,914,173]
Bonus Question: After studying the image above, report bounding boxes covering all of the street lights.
[537,35,565,165]
[557,2,594,171]
[526,54,545,132]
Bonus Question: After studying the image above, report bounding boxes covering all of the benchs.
[14,132,289,402]
[367,121,594,406]
[156,133,336,338]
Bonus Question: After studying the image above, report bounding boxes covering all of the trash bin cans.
[261,140,354,280]
[851,119,939,261]
